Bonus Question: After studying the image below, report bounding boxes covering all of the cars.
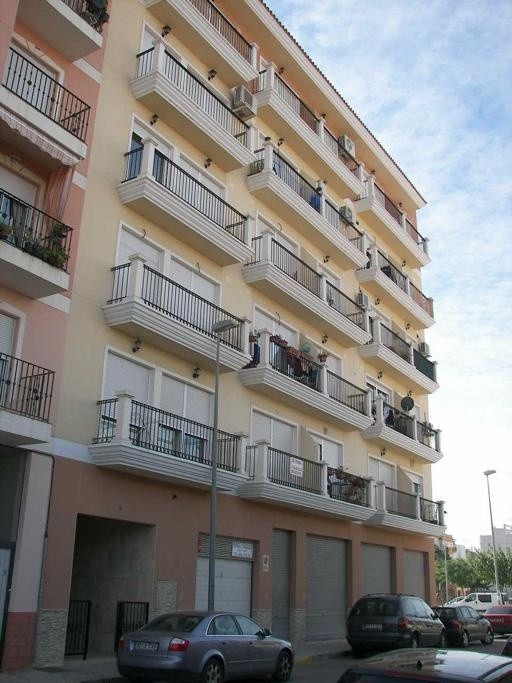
[117,609,295,683]
[332,591,512,683]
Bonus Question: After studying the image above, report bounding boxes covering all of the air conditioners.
[337,133,357,161]
[233,83,258,121]
[338,202,357,227]
[356,291,370,309]
[418,340,431,356]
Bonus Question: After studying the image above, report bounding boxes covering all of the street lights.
[484,469,503,607]
[205,319,239,614]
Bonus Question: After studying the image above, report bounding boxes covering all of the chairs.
[217,617,236,634]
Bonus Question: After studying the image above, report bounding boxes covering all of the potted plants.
[270,333,328,378]
[1,221,71,268]
